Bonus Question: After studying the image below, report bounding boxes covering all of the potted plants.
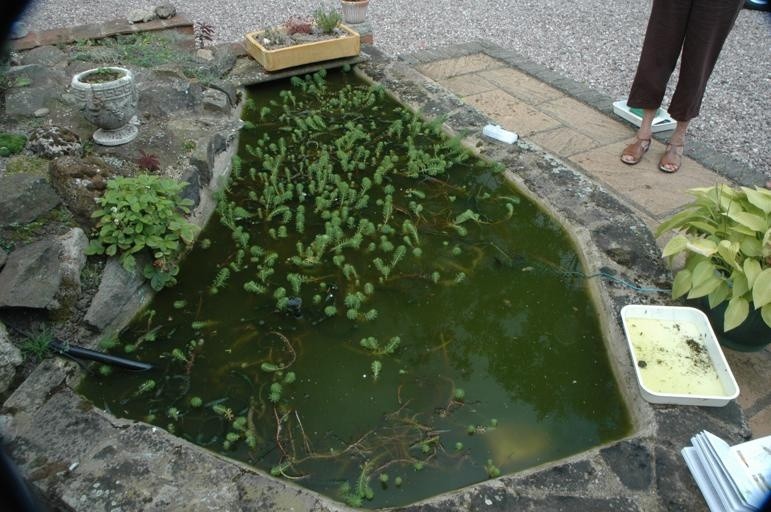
[655,184,771,352]
[246,9,360,71]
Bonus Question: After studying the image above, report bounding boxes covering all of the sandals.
[620,138,651,165]
[658,143,684,173]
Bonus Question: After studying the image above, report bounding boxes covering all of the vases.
[71,67,139,147]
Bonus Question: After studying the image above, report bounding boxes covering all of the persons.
[620,0,747,173]
